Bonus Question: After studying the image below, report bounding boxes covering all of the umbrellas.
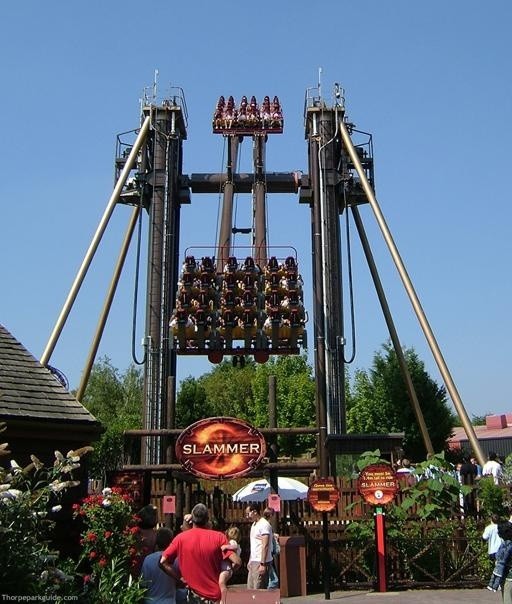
[231,474,311,531]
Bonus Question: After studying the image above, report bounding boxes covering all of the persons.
[487,529,511,592]
[482,514,502,590]
[171,256,302,347]
[499,524,512,603]
[214,94,282,129]
[395,451,511,519]
[134,504,282,604]
